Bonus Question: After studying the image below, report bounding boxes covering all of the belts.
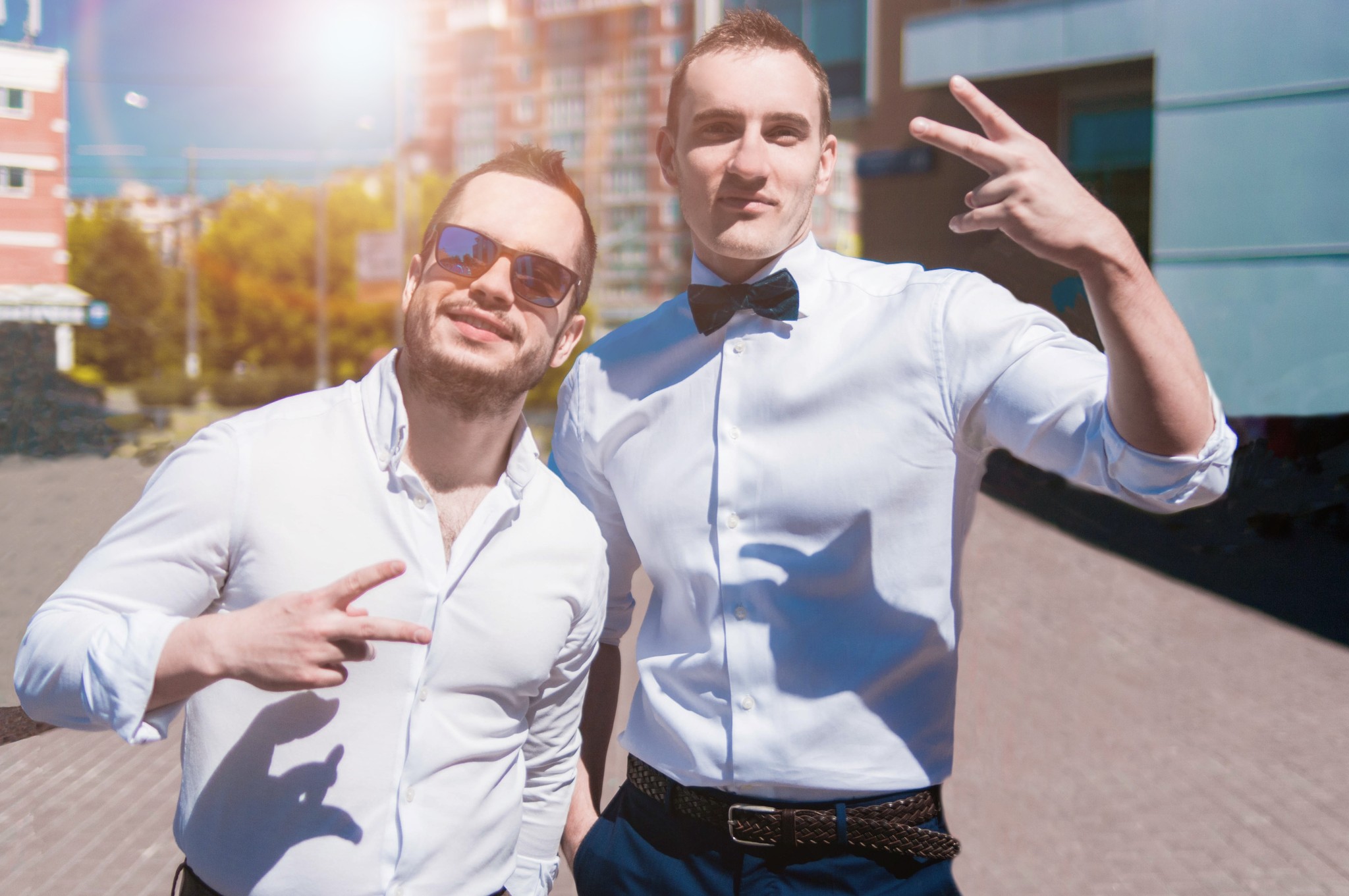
[622,756,960,858]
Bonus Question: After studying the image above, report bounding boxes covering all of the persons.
[548,6,1240,896]
[11,139,612,896]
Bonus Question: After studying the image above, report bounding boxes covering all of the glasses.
[420,226,582,309]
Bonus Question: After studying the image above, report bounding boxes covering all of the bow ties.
[686,267,801,335]
